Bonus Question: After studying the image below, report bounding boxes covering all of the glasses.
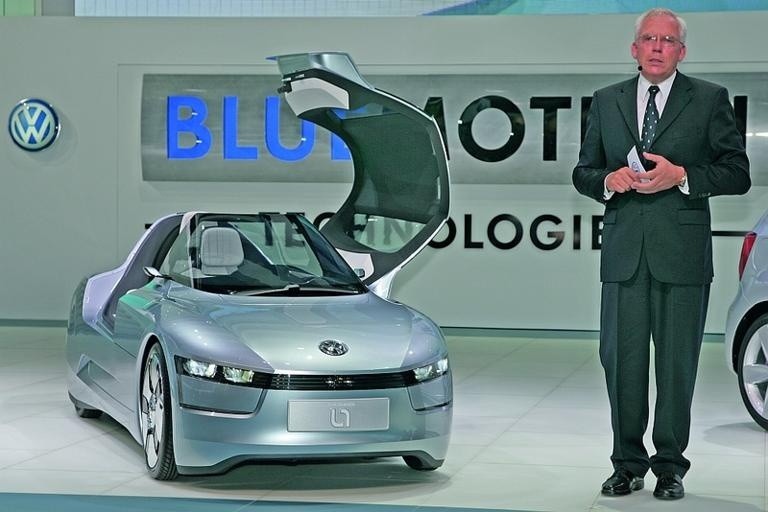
[638,33,683,45]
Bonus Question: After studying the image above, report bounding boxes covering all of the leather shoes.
[654,473,684,501]
[601,469,645,497]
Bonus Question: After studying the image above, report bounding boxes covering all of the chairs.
[181,227,244,278]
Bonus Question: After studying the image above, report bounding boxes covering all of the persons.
[571,9,751,501]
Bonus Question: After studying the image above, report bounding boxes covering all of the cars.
[66,52,453,480]
[724,211,768,430]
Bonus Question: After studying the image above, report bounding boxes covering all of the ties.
[640,85,660,155]
